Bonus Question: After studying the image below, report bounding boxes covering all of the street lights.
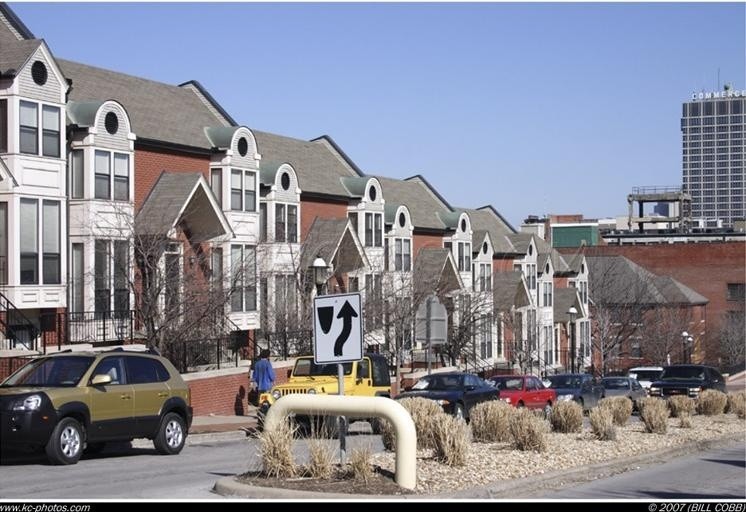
[308,253,331,295]
[565,306,579,374]
[681,332,694,365]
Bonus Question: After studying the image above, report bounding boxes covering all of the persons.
[248,350,276,407]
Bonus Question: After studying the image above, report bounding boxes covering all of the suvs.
[257,353,392,439]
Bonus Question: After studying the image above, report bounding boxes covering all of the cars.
[0,348,193,465]
